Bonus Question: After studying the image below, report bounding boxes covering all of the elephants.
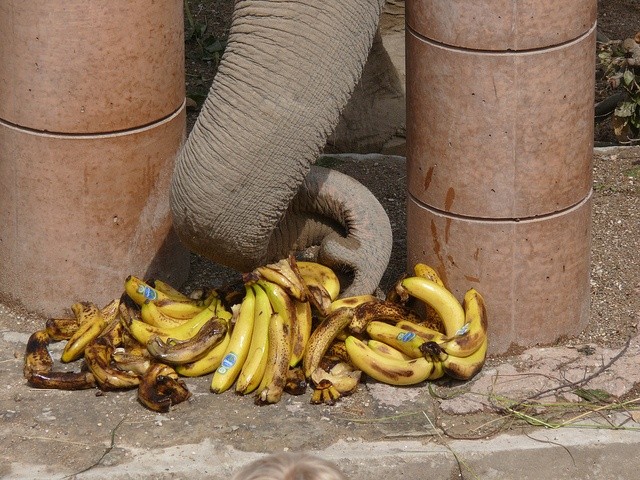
[168,0,408,300]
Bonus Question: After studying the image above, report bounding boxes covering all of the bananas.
[23,249,492,414]
